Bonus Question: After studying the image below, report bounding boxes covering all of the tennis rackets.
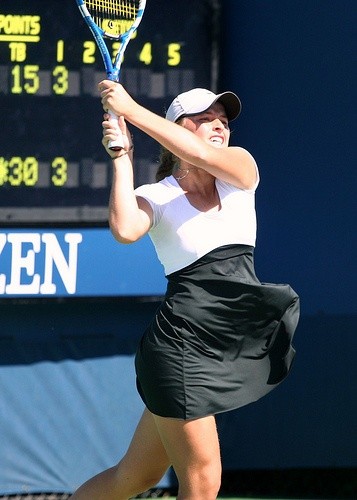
[76,0,146,151]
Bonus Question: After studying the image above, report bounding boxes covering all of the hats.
[166,88,241,127]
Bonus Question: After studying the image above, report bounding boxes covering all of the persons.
[68,79,260,500]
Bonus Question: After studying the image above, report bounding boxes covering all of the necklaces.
[175,167,202,181]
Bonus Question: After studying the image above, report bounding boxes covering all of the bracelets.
[110,144,135,160]
